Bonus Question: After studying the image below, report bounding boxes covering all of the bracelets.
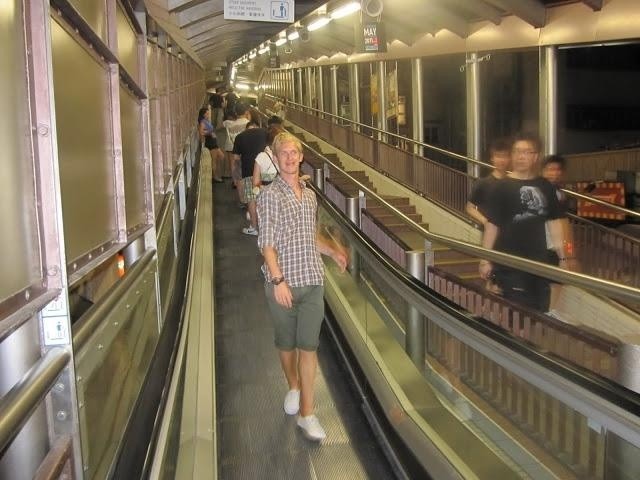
[271,274,285,285]
[557,256,567,262]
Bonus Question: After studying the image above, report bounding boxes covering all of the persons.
[540,154,579,327]
[197,84,287,237]
[465,143,512,280]
[255,131,348,439]
[478,132,570,396]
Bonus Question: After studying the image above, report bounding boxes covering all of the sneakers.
[242,227,258,235]
[297,414,326,440]
[283,389,301,415]
